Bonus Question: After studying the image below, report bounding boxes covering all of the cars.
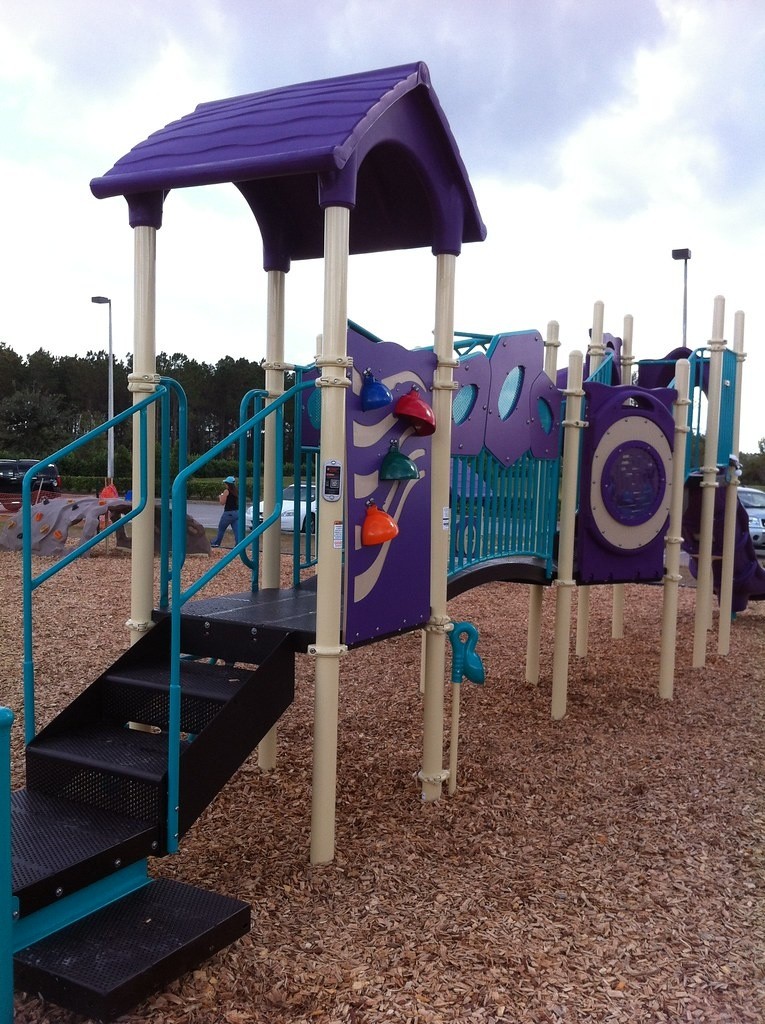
[245,482,317,536]
[737,486,765,548]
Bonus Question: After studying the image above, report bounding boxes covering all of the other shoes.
[210,541,218,546]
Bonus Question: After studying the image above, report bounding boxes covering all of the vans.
[0,458,62,512]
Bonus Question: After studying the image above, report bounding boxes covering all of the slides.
[681,467,765,612]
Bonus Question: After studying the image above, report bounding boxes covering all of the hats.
[223,476,235,483]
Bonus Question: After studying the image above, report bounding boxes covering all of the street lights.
[92,297,115,477]
[671,248,692,348]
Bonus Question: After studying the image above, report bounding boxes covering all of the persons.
[209,476,239,549]
